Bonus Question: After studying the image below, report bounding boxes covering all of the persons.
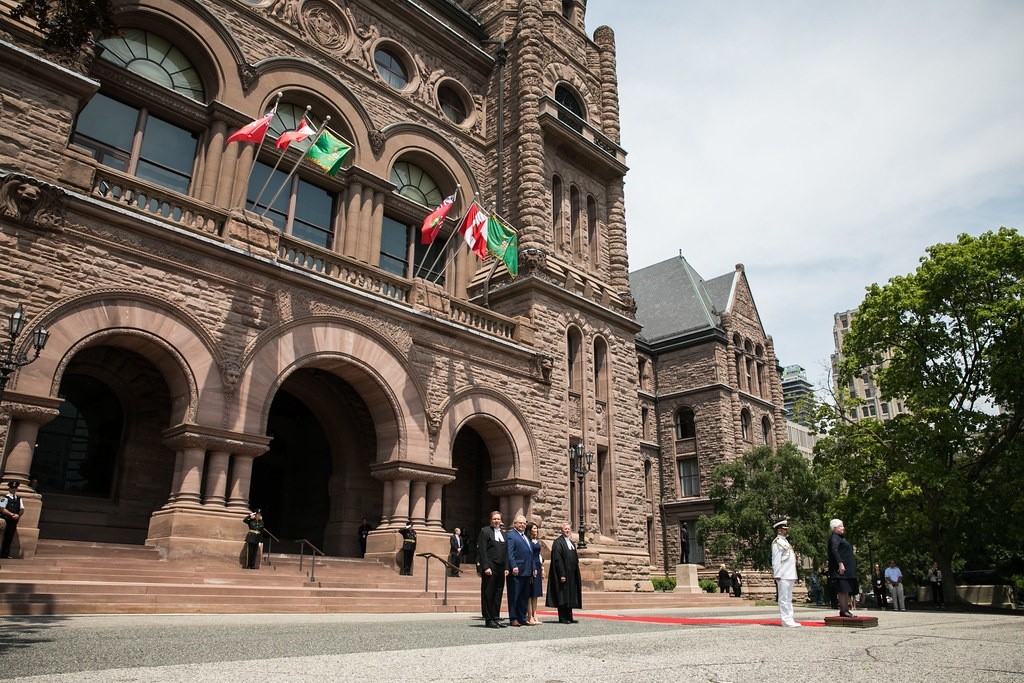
[0,481,25,559]
[544,523,582,624]
[718,563,742,597]
[477,510,543,629]
[450,527,470,577]
[30,479,37,490]
[827,519,859,617]
[928,561,946,609]
[872,560,905,611]
[243,508,264,569]
[679,522,690,563]
[398,522,417,575]
[771,520,802,628]
[358,518,372,557]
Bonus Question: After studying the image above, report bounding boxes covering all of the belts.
[249,529,260,534]
[404,539,416,542]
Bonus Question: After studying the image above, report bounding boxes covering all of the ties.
[522,533,525,540]
[13,494,15,500]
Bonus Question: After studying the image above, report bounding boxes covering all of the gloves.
[405,524,411,529]
[250,512,256,517]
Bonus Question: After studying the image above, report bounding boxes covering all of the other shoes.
[527,615,542,625]
[786,623,801,628]
[495,619,507,627]
[486,619,500,628]
[559,617,570,624]
[570,618,578,623]
[840,610,857,617]
[511,619,521,626]
[521,620,531,625]
[0,554,15,559]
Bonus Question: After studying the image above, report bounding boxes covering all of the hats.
[406,522,414,526]
[773,520,791,530]
[7,481,20,488]
[254,509,261,514]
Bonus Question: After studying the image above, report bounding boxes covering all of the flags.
[226,107,275,145]
[421,193,455,244]
[274,116,317,150]
[457,203,488,260]
[304,128,353,177]
[488,214,518,279]
